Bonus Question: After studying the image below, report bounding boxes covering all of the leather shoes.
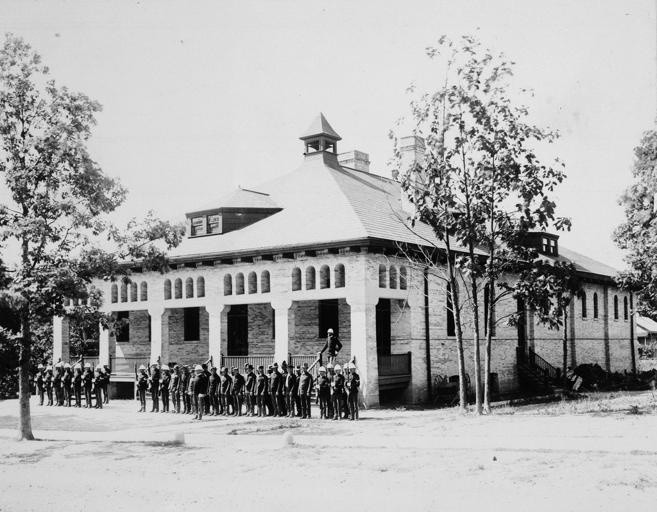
[138,408,358,421]
[38,402,109,409]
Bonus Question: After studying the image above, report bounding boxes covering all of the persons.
[317,329,342,362]
[37,362,111,409]
[138,362,360,420]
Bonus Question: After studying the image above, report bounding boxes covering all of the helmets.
[327,329,333,333]
[38,362,108,370]
[139,361,356,372]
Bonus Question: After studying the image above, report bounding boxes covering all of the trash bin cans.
[449,374,472,404]
[481,372,499,401]
